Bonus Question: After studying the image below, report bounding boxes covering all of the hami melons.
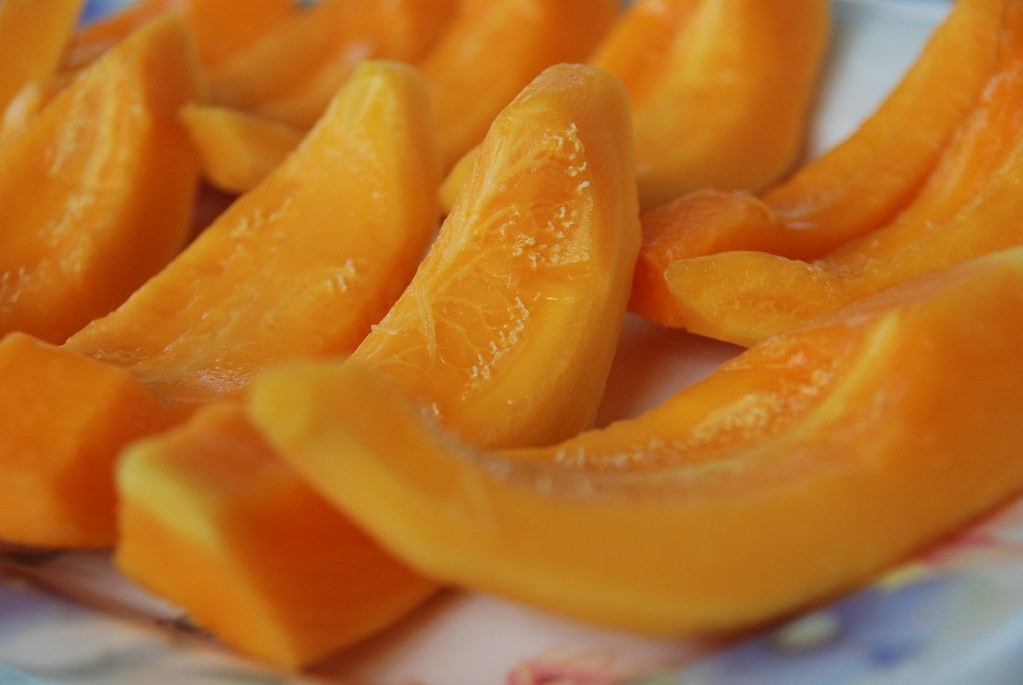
[0,0,1023,669]
